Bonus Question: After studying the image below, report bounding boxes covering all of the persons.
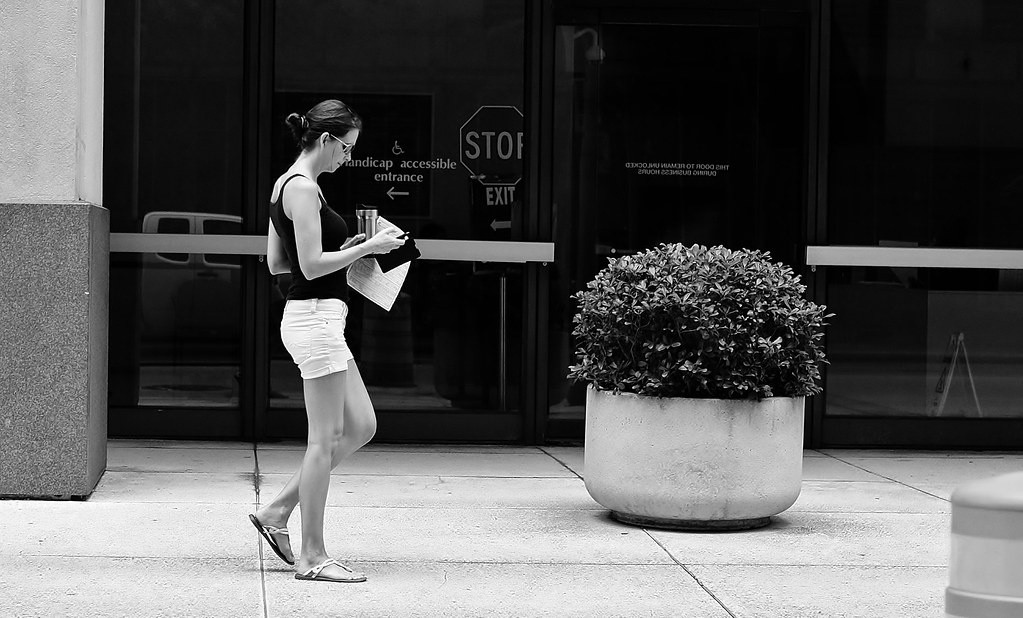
[247,96,406,583]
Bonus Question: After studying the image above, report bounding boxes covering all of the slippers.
[249,514,294,566]
[295,558,367,582]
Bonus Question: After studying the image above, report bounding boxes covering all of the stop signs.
[460,105,526,186]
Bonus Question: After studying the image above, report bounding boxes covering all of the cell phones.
[397,231,411,239]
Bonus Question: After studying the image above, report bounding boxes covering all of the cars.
[133,210,248,378]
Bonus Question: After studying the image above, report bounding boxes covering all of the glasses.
[329,134,353,155]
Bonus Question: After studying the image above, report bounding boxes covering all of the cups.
[356,206,378,243]
